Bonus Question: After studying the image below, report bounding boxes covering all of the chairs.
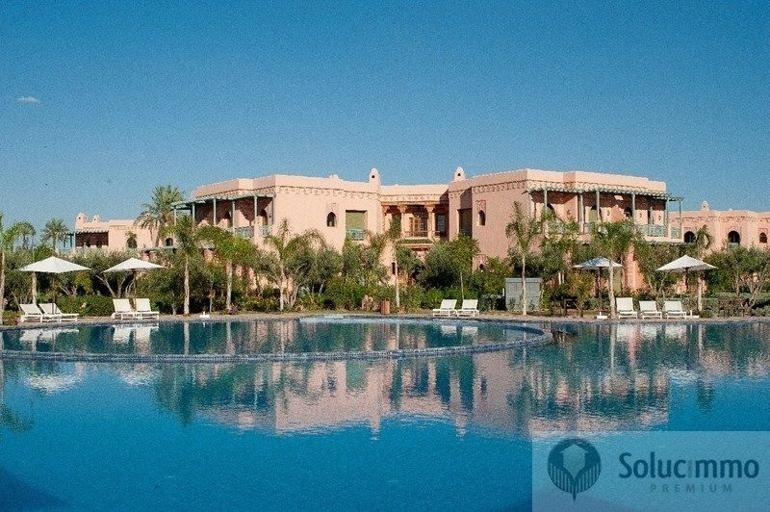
[615,294,689,320]
[17,296,160,323]
[431,298,480,320]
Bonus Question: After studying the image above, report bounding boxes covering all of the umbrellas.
[18,255,91,314]
[654,254,719,274]
[572,255,623,271]
[103,257,166,311]
